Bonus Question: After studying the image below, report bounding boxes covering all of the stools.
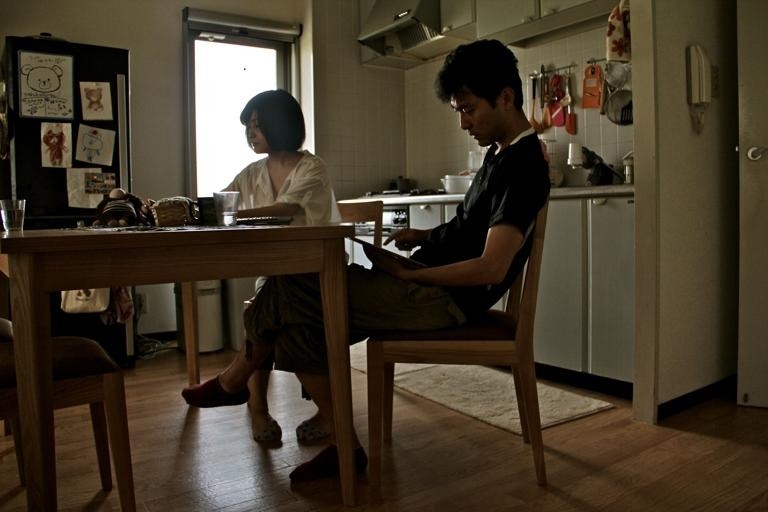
[0,335,139,511]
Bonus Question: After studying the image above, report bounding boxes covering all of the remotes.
[235,214,292,226]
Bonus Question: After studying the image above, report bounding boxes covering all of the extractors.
[354,0,468,70]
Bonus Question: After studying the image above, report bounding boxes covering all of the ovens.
[349,205,409,272]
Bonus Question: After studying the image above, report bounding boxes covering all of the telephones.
[688,43,714,107]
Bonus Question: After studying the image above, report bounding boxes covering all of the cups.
[211,190,241,227]
[566,140,584,167]
[0,196,27,234]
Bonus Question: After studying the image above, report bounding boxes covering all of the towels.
[604,3,632,63]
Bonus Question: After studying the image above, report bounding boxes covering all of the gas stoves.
[356,187,444,197]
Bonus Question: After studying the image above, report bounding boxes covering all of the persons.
[211,87,344,445]
[182,40,548,479]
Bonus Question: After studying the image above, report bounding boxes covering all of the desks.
[2,220,361,510]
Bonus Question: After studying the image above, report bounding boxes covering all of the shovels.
[542,73,551,128]
[621,100,633,124]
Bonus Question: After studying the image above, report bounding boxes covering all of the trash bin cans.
[174,281,224,354]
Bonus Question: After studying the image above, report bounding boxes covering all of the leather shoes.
[296,420,331,439]
[247,403,281,442]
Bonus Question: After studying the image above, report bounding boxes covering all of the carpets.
[345,339,615,436]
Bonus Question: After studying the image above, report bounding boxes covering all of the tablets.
[351,237,429,270]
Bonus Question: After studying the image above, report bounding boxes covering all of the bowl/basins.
[444,174,474,194]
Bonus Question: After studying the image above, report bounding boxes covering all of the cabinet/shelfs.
[439,0,619,45]
[404,202,458,231]
[503,196,634,382]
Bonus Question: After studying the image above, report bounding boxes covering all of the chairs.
[334,199,386,251]
[369,176,568,485]
